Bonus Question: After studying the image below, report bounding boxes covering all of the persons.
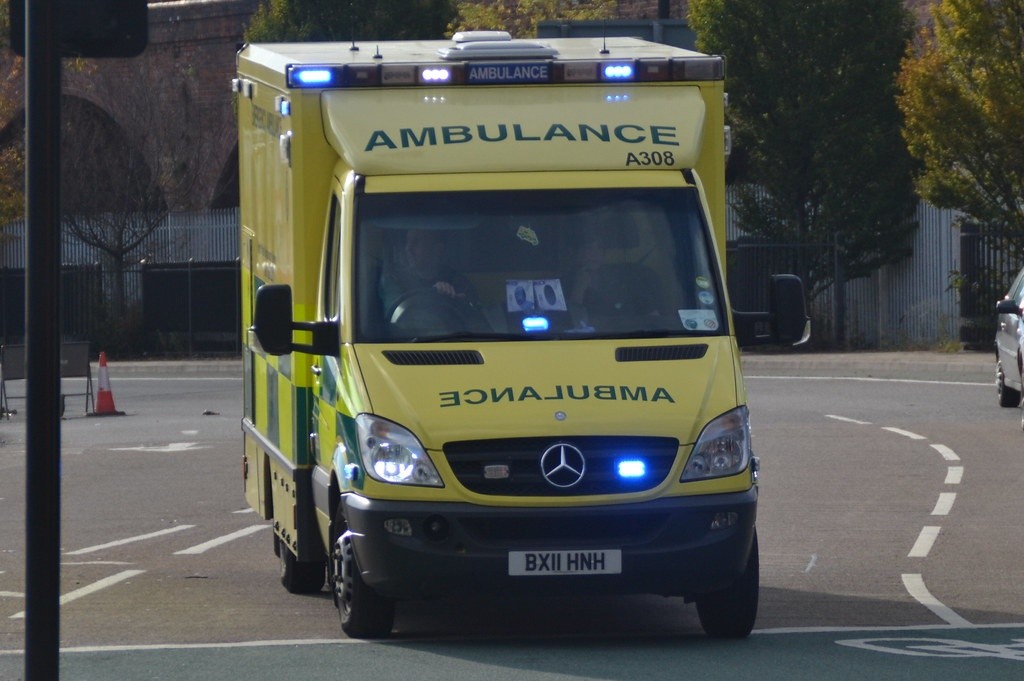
[392,217,486,339]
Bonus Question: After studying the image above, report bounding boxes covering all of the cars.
[993,264,1024,408]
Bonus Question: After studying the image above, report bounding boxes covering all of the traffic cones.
[83,351,126,417]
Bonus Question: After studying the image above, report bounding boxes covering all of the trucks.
[231,18,812,641]
